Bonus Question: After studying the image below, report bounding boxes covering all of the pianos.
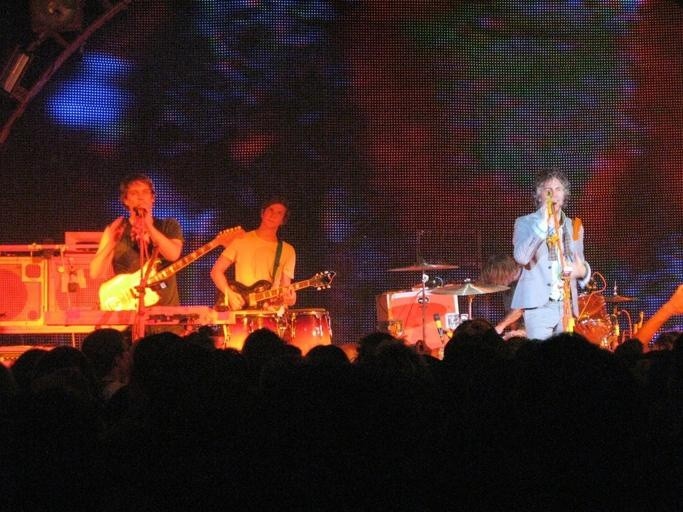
[144,307,211,326]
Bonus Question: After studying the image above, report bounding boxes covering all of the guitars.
[98,225,243,332]
[214,269,335,311]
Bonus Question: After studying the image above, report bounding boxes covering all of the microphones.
[433,313,446,344]
[136,200,148,230]
[542,193,553,215]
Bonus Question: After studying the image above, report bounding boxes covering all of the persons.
[88,174,184,310]
[209,199,296,312]
[479,250,527,340]
[511,169,592,346]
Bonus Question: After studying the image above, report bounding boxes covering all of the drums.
[224,307,330,357]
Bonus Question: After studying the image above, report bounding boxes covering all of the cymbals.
[391,264,456,273]
[606,295,634,303]
[432,285,510,295]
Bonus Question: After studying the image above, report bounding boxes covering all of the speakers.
[0,256,47,329]
[377,286,461,357]
[48,255,130,327]
